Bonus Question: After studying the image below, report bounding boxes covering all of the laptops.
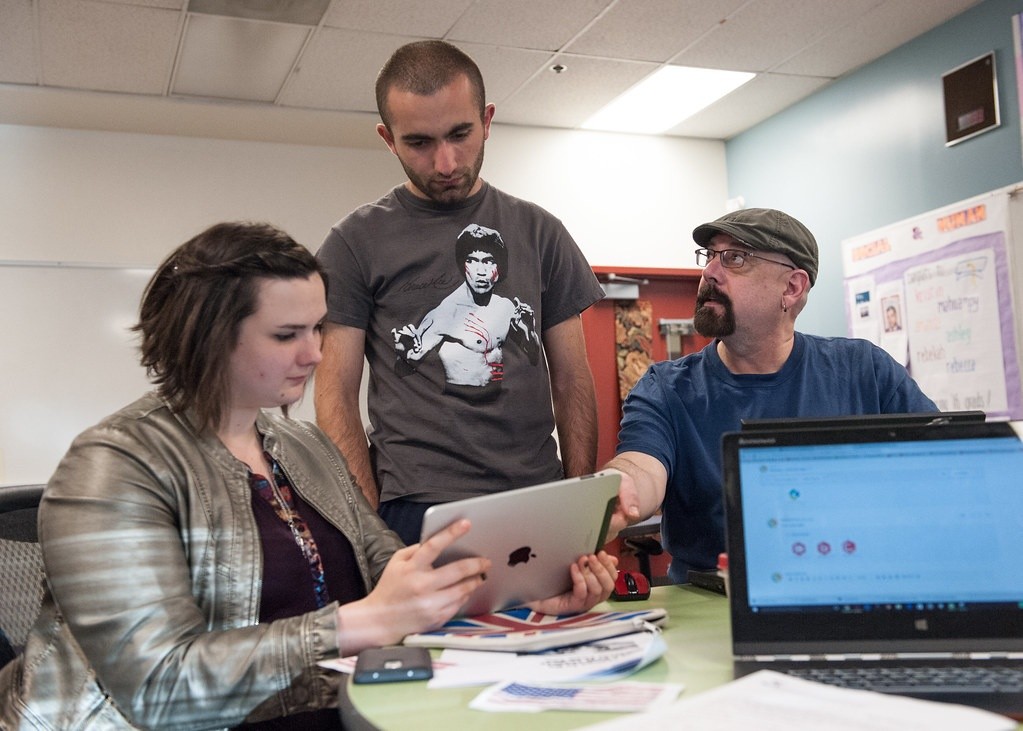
[719,422,1023,712]
[685,410,986,596]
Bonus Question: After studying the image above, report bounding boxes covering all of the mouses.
[611,570,651,602]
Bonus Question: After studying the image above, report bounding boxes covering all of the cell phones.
[353,645,434,685]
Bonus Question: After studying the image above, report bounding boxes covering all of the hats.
[691,208,819,287]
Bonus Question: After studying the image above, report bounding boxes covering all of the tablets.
[420,468,622,617]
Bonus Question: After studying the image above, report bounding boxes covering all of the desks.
[335,582,738,731]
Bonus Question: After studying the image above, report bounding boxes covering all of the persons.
[604,208,942,597]
[1,221,619,731]
[313,40,603,547]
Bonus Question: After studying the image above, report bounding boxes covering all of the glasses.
[695,249,793,267]
